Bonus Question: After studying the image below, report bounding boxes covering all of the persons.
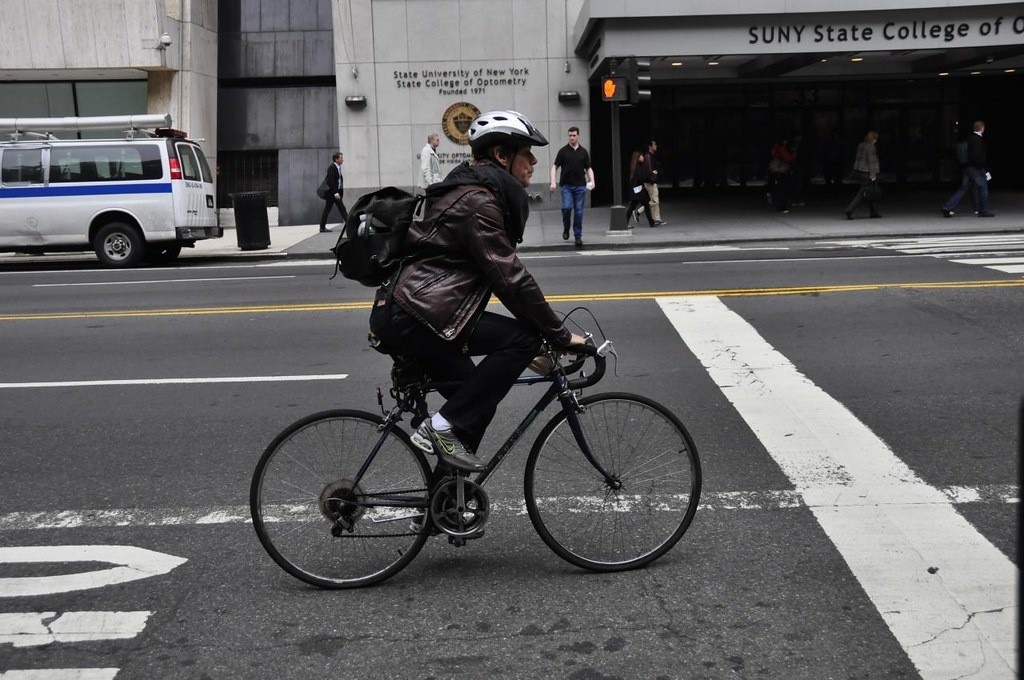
[417,132,443,195]
[766,132,805,214]
[627,140,666,229]
[550,127,595,245]
[941,120,994,217]
[367,110,586,533]
[319,152,348,232]
[844,131,880,218]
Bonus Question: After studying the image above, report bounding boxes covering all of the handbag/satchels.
[770,151,788,174]
[864,180,879,202]
[317,182,332,200]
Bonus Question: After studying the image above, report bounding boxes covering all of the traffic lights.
[601,75,631,103]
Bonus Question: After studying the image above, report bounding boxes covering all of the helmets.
[466,110,550,147]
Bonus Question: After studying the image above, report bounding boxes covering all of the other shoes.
[320,227,333,233]
[870,213,881,217]
[562,228,570,240]
[575,236,583,246]
[977,211,994,217]
[844,210,855,219]
[652,221,661,226]
[628,225,633,229]
[941,208,952,216]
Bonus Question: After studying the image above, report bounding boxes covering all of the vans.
[0,114,224,269]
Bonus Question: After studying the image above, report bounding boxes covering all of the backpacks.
[332,180,499,287]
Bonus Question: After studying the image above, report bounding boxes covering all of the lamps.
[558,91,578,102]
[345,96,367,107]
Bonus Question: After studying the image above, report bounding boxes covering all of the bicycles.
[249,305,703,591]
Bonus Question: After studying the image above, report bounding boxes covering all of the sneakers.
[409,417,485,473]
[632,209,640,224]
[409,506,476,534]
[655,219,668,225]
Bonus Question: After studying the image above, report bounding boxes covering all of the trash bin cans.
[229,191,272,250]
